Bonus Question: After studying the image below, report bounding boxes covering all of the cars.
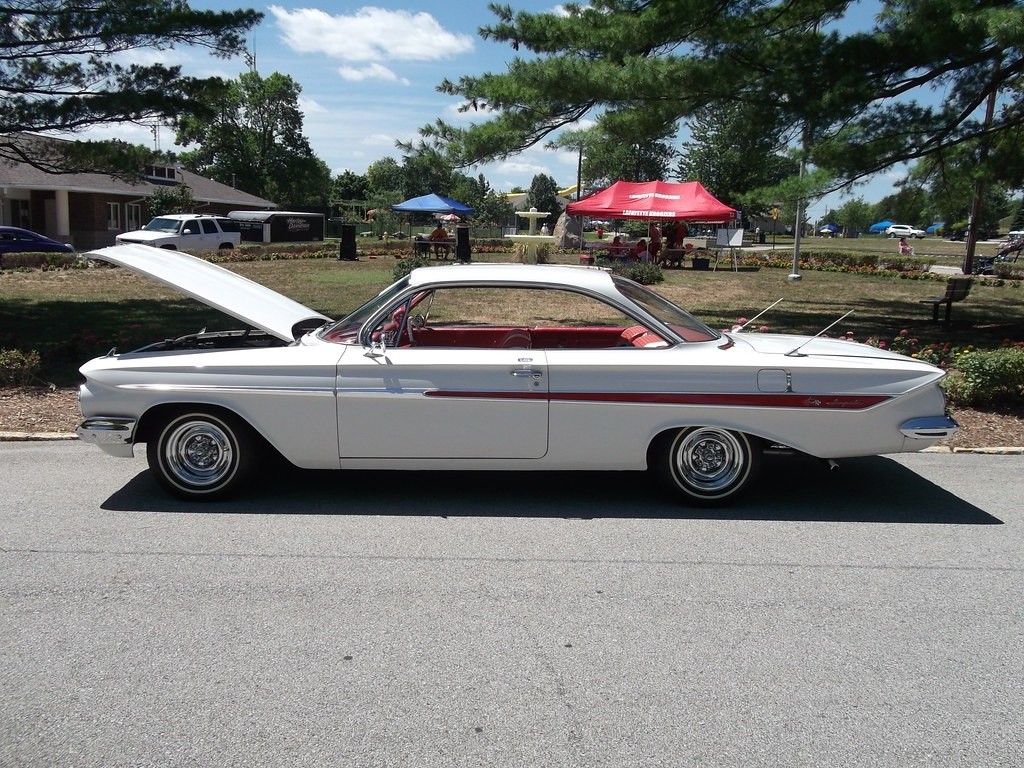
[887,225,926,239]
[845,231,860,238]
[75,242,960,507]
[1008,227,1024,242]
[950,228,987,242]
[0,226,76,253]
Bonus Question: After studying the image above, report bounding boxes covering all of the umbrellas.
[819,224,837,233]
[869,220,895,233]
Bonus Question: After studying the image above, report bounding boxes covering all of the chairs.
[617,326,664,349]
[498,329,537,349]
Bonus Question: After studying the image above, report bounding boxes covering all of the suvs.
[116,213,242,250]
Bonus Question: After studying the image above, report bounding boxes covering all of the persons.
[598,228,603,239]
[610,223,685,262]
[899,238,912,254]
[430,223,450,260]
[541,223,549,235]
[756,226,760,233]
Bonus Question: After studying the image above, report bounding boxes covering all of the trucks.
[227,210,324,243]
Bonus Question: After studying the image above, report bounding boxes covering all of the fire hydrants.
[382,231,389,243]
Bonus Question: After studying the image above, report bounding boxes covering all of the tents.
[566,181,735,264]
[392,193,476,234]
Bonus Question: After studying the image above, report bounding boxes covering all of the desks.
[413,241,456,259]
[586,245,636,264]
[669,247,741,272]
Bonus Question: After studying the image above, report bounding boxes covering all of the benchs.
[920,278,974,324]
